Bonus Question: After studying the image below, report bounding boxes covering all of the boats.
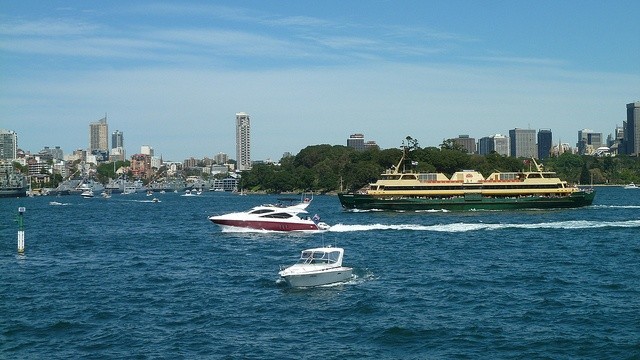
[279,247,353,288]
[209,189,331,231]
[153,197,159,203]
[144,189,202,197]
[81,189,95,198]
[624,181,638,190]
[100,193,112,199]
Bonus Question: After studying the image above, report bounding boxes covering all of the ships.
[337,146,596,210]
